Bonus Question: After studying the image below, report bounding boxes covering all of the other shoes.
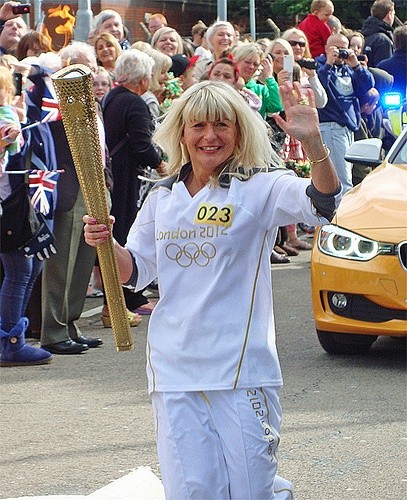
[270,250,290,263]
[86,289,103,298]
[134,302,154,315]
[274,245,299,256]
[291,241,313,250]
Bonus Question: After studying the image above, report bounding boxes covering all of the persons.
[83,80,343,500]
[0,0,407,367]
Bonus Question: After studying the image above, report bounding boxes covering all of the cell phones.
[13,3,31,15]
[12,73,23,95]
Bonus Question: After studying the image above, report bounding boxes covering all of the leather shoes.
[40,339,89,354]
[72,336,103,348]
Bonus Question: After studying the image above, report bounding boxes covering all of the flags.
[26,170,60,220]
[42,80,62,124]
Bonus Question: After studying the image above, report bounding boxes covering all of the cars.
[312,125,407,355]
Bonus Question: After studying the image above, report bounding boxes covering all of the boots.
[0,318,53,366]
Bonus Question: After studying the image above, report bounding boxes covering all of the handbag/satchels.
[0,184,39,253]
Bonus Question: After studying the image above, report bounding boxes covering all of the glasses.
[288,40,306,47]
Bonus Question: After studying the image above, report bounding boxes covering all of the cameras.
[357,55,365,61]
[337,49,349,60]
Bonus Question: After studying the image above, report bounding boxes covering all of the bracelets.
[307,144,330,165]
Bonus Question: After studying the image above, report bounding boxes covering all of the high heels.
[100,309,142,328]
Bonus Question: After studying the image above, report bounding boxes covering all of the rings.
[298,97,306,104]
